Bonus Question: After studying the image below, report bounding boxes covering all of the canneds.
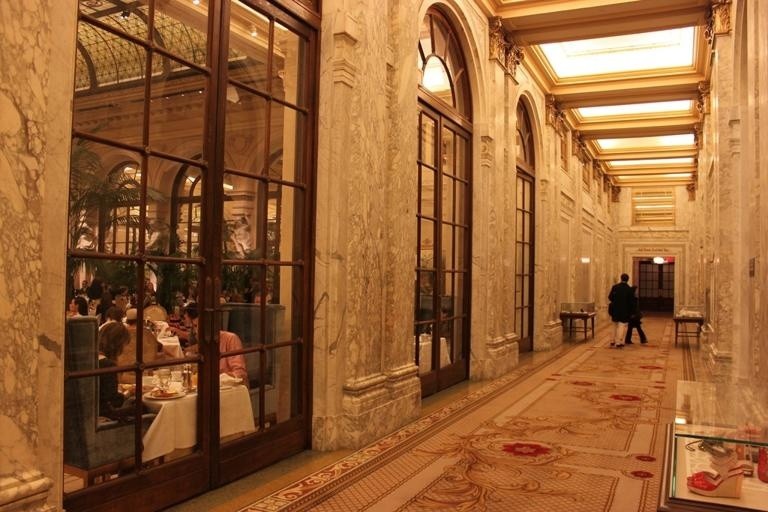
[182,370,192,387]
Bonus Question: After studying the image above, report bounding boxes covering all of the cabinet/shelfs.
[657,379,768,512]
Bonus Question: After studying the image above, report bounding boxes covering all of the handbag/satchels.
[607,302,613,316]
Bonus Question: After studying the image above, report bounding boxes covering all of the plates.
[144,391,185,399]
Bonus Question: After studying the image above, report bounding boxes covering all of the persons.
[66,281,249,417]
[626,285,649,344]
[608,273,632,346]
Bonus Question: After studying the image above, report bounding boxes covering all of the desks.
[673,314,704,345]
[560,311,597,343]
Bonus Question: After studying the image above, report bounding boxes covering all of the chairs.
[65,315,158,489]
[226,308,279,428]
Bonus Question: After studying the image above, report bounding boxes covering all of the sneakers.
[609,342,614,346]
[624,342,634,345]
[640,341,648,343]
[615,345,624,347]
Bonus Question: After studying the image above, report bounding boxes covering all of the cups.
[143,368,192,392]
